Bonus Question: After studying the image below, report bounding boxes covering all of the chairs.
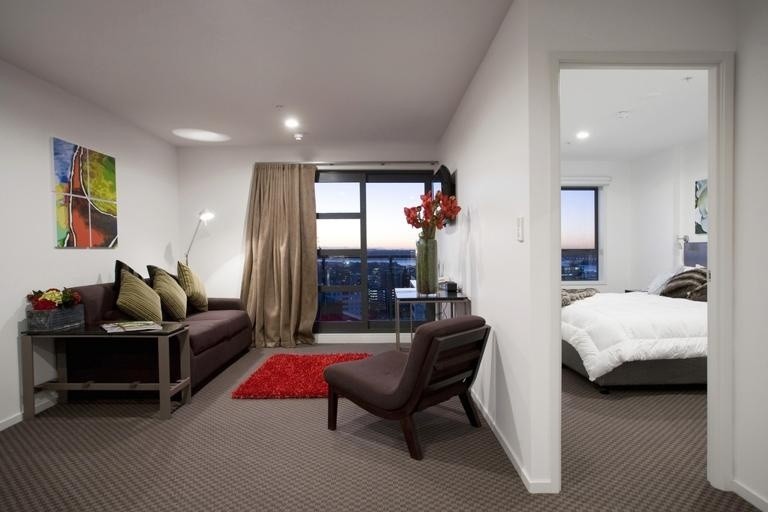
[325,316,491,460]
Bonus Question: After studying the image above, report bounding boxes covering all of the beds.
[562,292,707,395]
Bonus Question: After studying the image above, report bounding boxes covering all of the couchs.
[63,278,251,404]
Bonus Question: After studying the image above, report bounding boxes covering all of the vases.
[416,230,437,294]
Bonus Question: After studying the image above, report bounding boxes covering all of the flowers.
[25,287,81,309]
[403,191,462,228]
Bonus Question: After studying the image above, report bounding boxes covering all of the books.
[100,319,163,334]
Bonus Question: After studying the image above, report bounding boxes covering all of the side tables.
[21,322,191,421]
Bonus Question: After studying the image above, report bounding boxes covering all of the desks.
[393,287,471,352]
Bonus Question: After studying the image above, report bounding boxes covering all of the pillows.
[146,263,180,321]
[154,269,187,321]
[176,261,207,312]
[117,269,162,323]
[113,260,145,320]
[660,269,707,301]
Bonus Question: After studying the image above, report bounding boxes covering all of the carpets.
[232,353,371,399]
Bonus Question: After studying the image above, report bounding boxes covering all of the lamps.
[184,208,215,265]
